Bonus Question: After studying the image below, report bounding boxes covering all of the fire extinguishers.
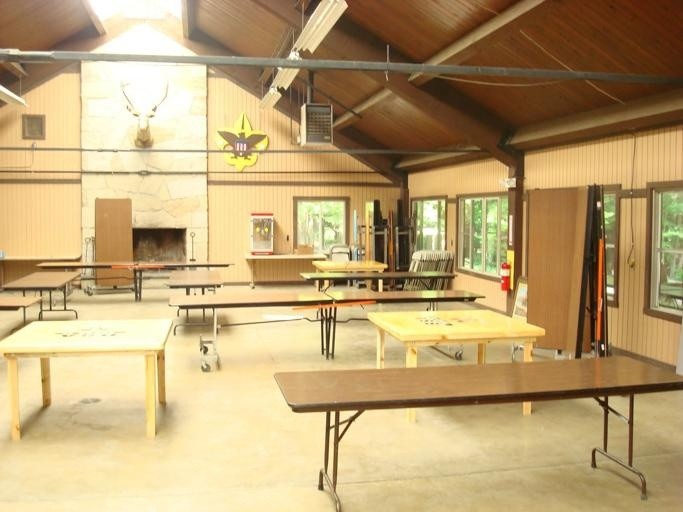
[501,263,510,291]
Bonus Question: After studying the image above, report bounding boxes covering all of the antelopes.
[119,83,170,148]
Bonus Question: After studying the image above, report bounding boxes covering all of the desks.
[272,355,683,512]
[247,252,331,291]
[37,261,234,320]
[0,319,173,439]
[302,260,487,359]
[3,271,83,323]
[368,310,546,422]
[169,293,334,360]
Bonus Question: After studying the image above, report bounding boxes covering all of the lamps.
[258,0,349,114]
[1,60,27,110]
